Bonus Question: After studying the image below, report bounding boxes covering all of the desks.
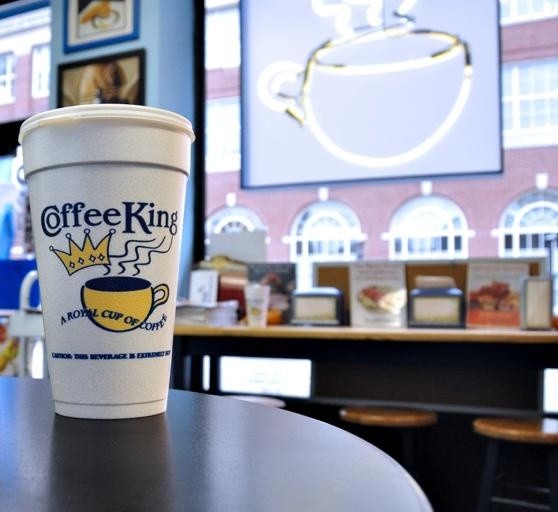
[0,373,432,512]
[171,312,557,422]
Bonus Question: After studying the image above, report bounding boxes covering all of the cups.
[243,284,270,328]
[19,103,197,420]
[269,28,468,164]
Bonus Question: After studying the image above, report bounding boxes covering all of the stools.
[224,394,286,409]
[468,417,557,512]
[337,403,436,430]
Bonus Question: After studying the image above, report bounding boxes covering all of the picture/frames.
[60,0,140,55]
[55,48,146,106]
